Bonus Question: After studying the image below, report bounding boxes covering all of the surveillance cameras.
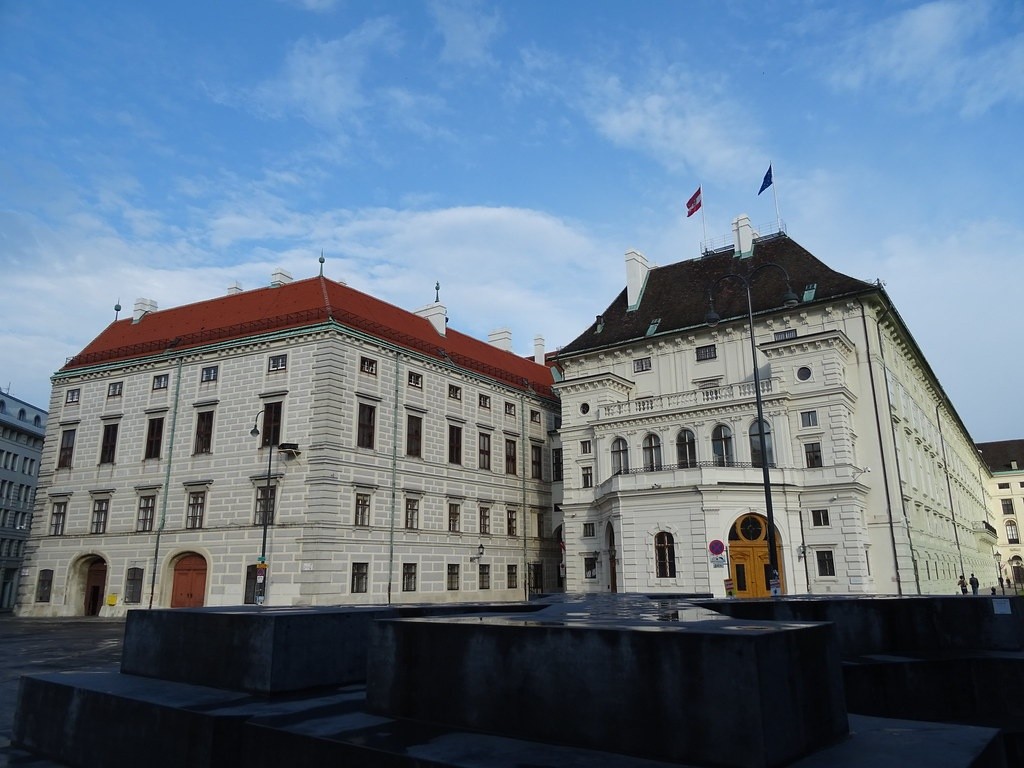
[864,465,872,473]
[833,495,837,501]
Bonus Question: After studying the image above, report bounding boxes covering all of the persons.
[1006,577,1011,587]
[969,574,979,594]
[958,576,968,594]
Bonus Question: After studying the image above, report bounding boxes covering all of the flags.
[758,165,773,195]
[686,187,702,217]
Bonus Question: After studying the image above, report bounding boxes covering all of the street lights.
[1006,557,1018,596]
[1014,561,1024,592]
[993,550,1004,595]
[247,400,275,611]
[699,264,799,597]
